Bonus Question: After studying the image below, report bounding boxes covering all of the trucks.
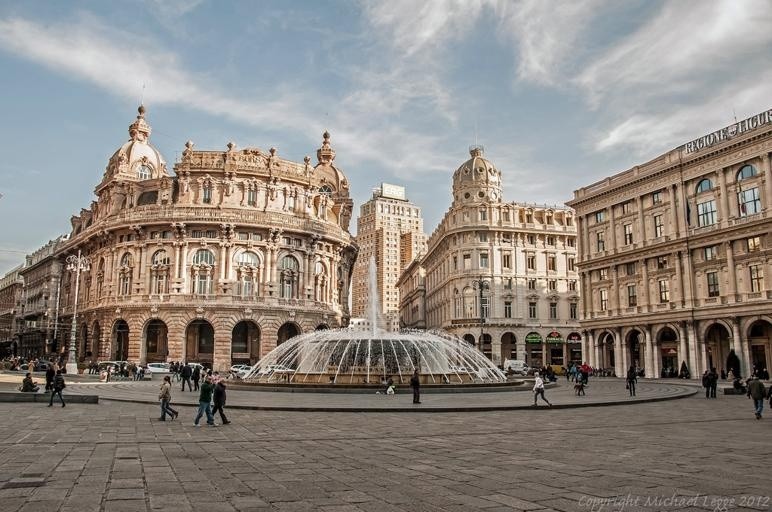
[504,356,530,376]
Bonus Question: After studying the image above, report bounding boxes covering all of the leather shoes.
[176,411,179,418]
[172,413,174,420]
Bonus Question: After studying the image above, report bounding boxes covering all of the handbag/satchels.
[62,380,66,389]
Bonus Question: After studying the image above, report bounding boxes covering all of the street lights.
[472,273,491,354]
[64,248,93,375]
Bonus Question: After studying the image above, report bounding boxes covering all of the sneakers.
[191,421,232,427]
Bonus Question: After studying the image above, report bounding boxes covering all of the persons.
[410,368,422,404]
[532,371,554,410]
[48,369,66,408]
[746,372,766,420]
[382,379,397,395]
[163,375,178,420]
[765,381,771,412]
[0,353,238,394]
[208,378,231,424]
[193,375,219,427]
[159,377,174,423]
[497,358,771,399]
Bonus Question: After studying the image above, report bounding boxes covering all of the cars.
[230,365,296,374]
[488,367,508,377]
[146,362,210,373]
[449,365,468,372]
[97,361,132,375]
